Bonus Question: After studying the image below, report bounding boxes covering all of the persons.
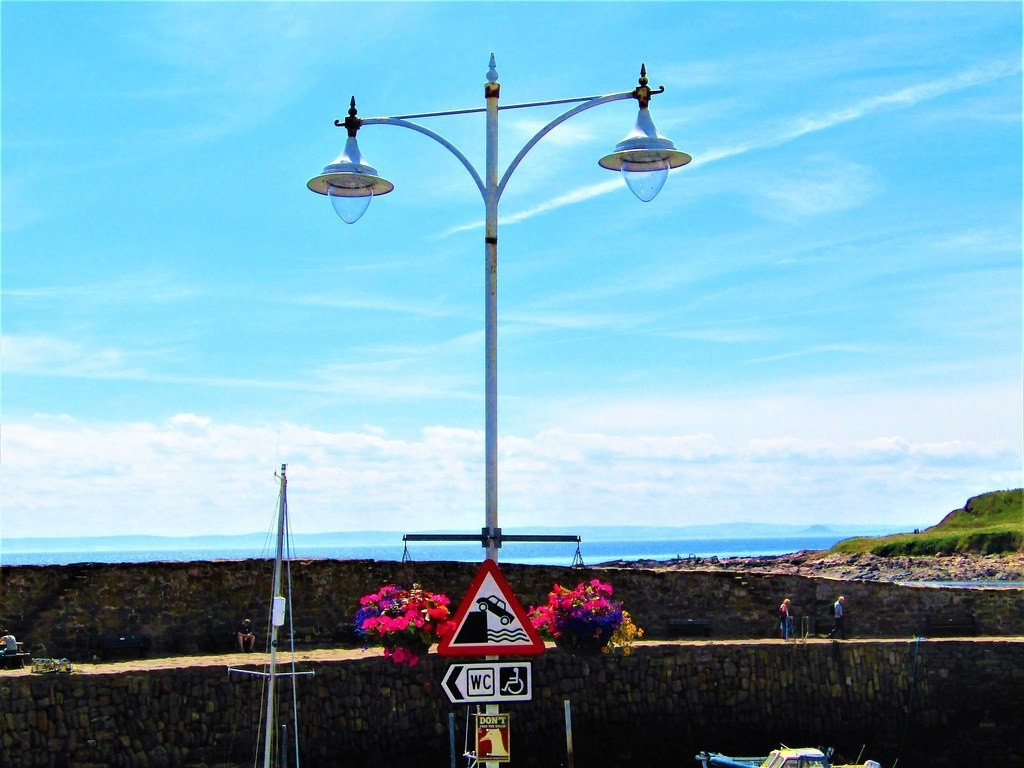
[780,599,794,639]
[827,596,848,640]
[238,619,255,653]
[0,630,18,655]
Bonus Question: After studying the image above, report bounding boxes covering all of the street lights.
[304,49,694,768]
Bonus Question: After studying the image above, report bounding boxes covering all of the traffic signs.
[440,661,531,704]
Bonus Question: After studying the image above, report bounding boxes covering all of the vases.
[553,623,616,655]
[385,632,434,658]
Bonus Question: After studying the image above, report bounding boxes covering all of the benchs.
[924,615,977,637]
[669,618,713,637]
[98,635,148,659]
[814,619,850,637]
[16,653,31,656]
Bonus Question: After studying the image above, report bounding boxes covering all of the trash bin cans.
[800,616,815,637]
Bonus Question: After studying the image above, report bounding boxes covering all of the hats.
[784,599,791,604]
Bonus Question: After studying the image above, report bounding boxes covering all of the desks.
[0,642,25,669]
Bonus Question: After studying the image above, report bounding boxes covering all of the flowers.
[350,581,451,668]
[528,580,643,657]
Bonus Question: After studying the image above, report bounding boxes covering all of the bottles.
[32,657,72,672]
